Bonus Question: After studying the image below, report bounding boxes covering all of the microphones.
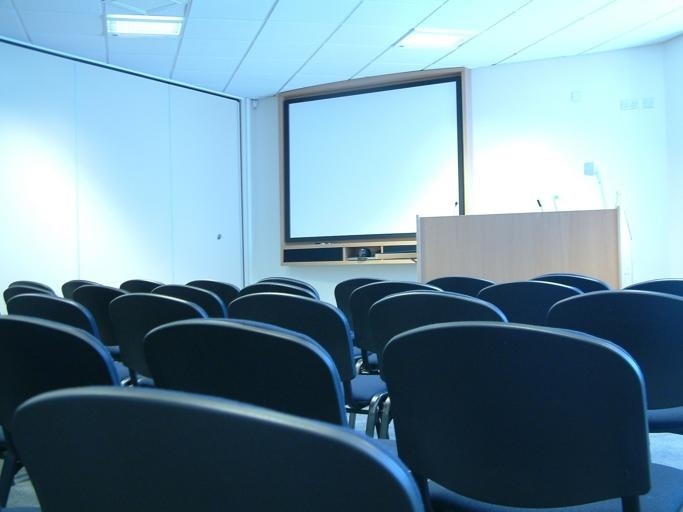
[537,199,545,211]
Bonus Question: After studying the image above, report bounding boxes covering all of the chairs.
[0,278,682,510]
